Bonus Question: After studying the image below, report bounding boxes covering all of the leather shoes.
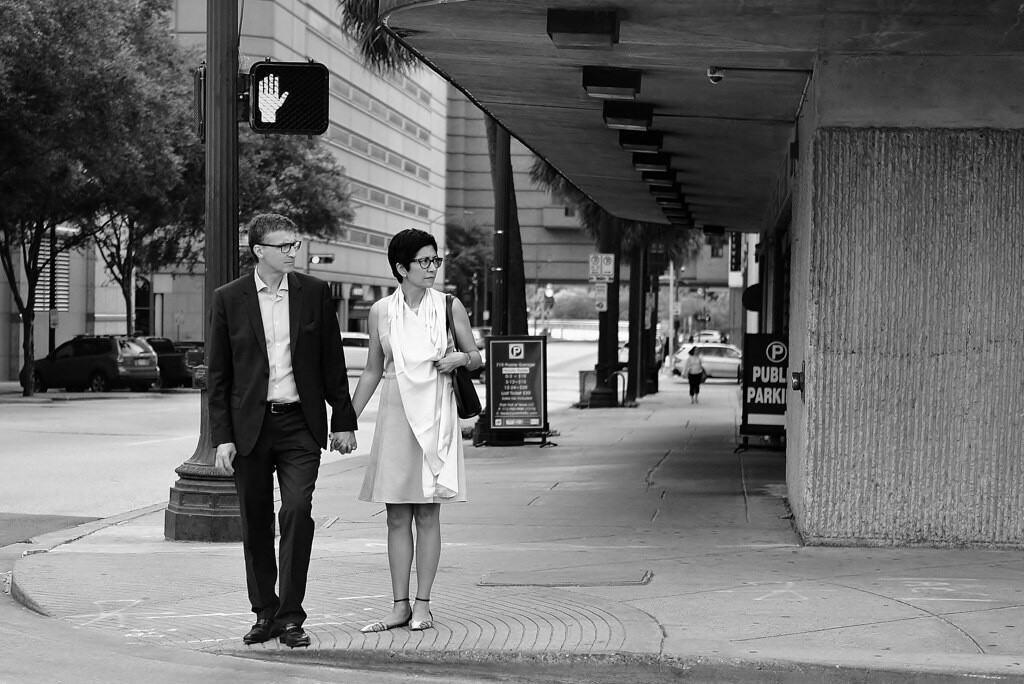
[243,615,281,643]
[280,620,310,647]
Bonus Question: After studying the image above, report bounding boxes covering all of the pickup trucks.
[136,336,186,392]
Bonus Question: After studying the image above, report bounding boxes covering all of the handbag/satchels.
[445,293,483,420]
[701,370,706,382]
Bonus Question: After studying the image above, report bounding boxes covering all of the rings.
[440,371,442,374]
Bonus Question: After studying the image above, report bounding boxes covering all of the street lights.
[669,260,685,377]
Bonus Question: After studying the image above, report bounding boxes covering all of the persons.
[206,214,359,645]
[682,348,703,403]
[328,230,481,634]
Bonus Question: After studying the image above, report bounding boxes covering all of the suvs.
[19,335,160,392]
[689,329,727,343]
[673,342,742,381]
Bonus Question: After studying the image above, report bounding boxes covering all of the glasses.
[410,257,443,268]
[255,240,302,253]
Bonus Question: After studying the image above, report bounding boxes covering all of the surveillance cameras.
[706,70,725,84]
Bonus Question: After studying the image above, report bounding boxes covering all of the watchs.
[464,351,471,368]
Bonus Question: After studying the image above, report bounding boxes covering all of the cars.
[173,342,204,353]
[340,331,369,369]
[471,327,493,381]
[618,336,664,369]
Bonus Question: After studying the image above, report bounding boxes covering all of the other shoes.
[361,598,412,633]
[408,597,433,630]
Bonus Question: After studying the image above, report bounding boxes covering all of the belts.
[265,399,301,414]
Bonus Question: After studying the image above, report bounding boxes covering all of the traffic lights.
[249,62,329,134]
[311,256,333,263]
